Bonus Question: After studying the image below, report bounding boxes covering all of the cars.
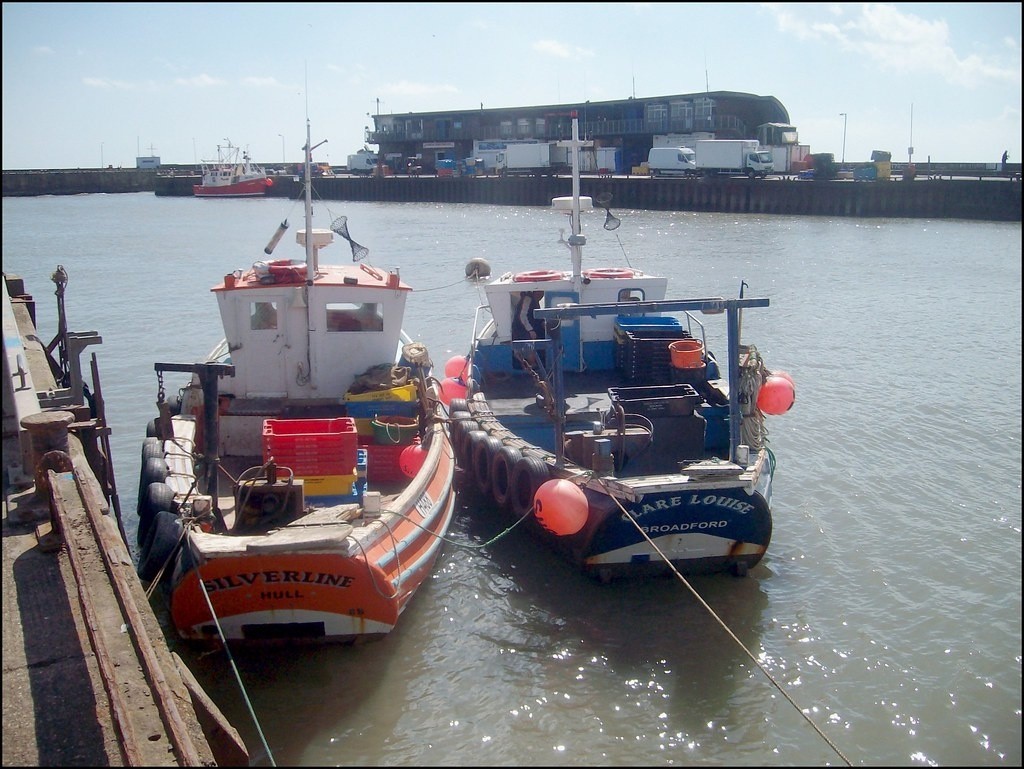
[265,166,288,176]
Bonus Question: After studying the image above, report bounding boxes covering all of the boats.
[441,111,798,587]
[191,137,273,197]
[132,118,461,645]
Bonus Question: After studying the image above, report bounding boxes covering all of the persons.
[514,292,545,368]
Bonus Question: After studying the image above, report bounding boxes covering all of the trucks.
[495,143,568,175]
[693,138,776,178]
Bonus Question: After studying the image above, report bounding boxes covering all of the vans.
[648,146,701,176]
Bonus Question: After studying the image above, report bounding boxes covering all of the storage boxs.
[608,315,700,417]
[262,378,420,520]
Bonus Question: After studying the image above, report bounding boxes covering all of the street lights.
[278,134,285,167]
[839,113,848,163]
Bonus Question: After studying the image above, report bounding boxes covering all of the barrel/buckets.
[669,362,706,394]
[371,415,418,445]
[668,339,704,368]
[902,163,915,181]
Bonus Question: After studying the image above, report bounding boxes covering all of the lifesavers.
[446,397,485,470]
[468,437,500,492]
[510,458,548,527]
[582,267,634,279]
[511,270,564,281]
[135,415,182,581]
[254,259,308,283]
[488,448,519,510]
